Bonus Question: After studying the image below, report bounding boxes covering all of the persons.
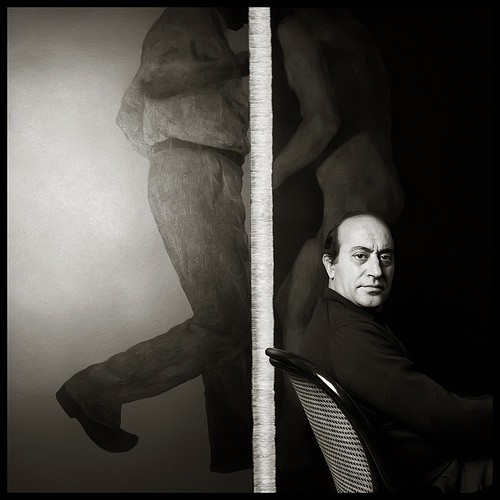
[298,211,500,499]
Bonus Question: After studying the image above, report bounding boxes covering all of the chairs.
[265,347,392,494]
